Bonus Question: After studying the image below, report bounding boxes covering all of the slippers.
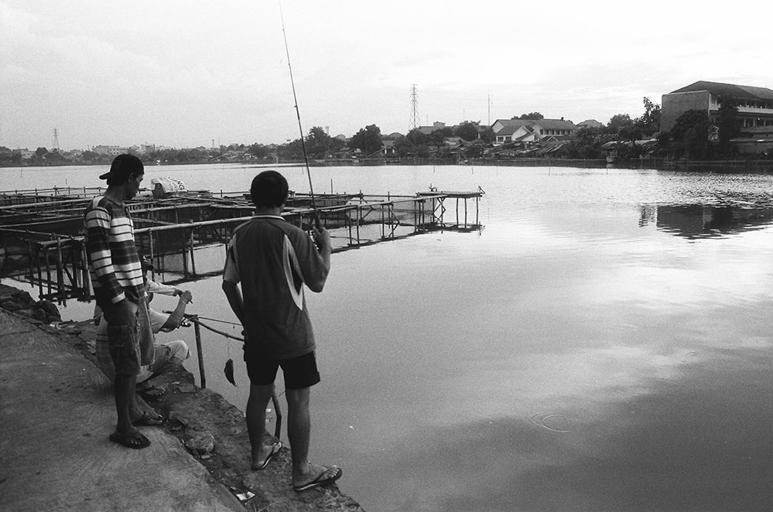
[109,430,151,449]
[132,410,165,426]
[293,464,343,492]
[136,385,165,399]
[259,440,284,470]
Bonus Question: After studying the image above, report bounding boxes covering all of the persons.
[95,251,191,384]
[81,152,168,449]
[222,169,342,493]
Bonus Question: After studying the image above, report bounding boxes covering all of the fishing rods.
[164,312,245,341]
[280,1,322,233]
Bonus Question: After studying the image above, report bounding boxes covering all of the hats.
[138,253,154,271]
[99,154,144,180]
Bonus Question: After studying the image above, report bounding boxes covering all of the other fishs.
[224,358,238,387]
